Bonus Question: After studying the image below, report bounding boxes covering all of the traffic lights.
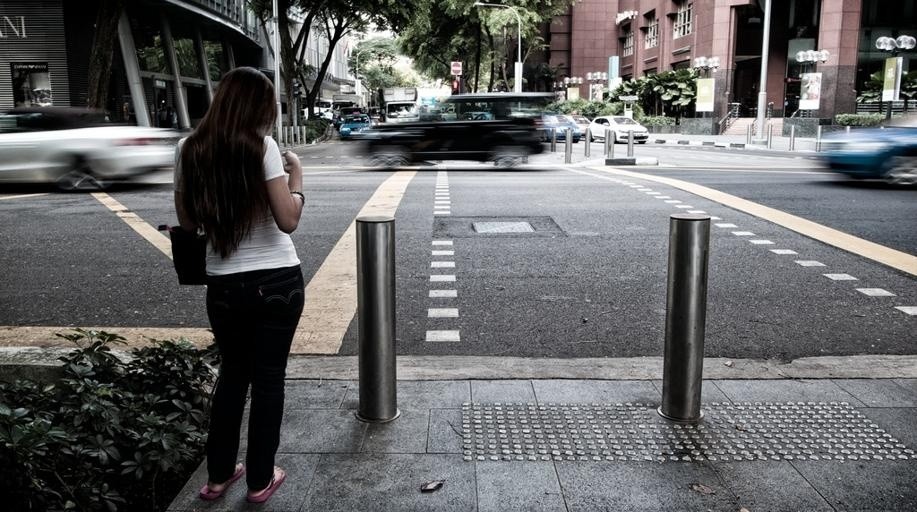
[292,79,302,99]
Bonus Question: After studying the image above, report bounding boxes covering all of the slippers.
[199,466,245,503]
[247,468,285,503]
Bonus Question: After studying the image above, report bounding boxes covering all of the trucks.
[378,88,420,123]
[333,95,365,128]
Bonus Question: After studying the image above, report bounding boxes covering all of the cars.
[818,108,917,189]
[364,91,544,171]
[0,106,181,193]
[338,107,372,140]
[542,115,650,143]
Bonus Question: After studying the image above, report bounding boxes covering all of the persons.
[173,67,304,502]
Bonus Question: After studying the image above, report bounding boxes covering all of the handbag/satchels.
[158,225,207,285]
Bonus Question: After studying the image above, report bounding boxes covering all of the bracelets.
[290,192,304,207]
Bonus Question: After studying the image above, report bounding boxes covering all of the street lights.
[473,1,522,93]
[875,34,917,120]
[692,55,719,118]
[552,71,610,104]
[796,49,829,117]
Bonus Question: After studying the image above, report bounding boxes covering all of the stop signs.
[451,61,462,76]
